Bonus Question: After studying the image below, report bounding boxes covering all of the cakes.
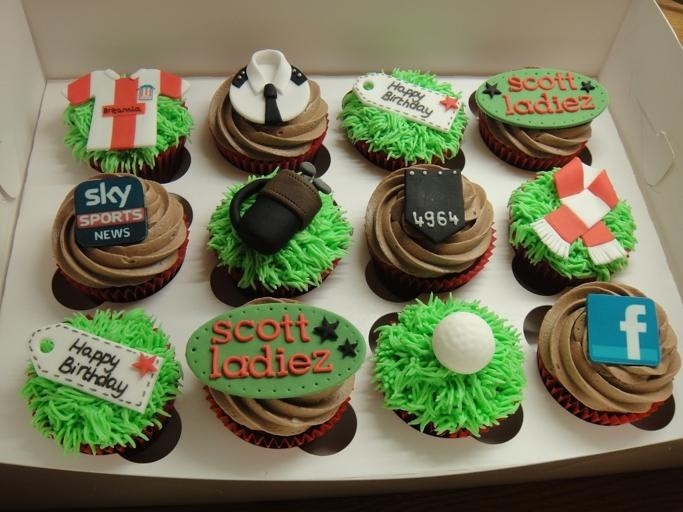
[369,293,528,444]
[50,173,193,309]
[523,281,681,431]
[185,297,366,456]
[364,164,497,302]
[470,66,609,170]
[337,69,469,173]
[507,156,638,298]
[206,162,353,306]
[207,49,331,178]
[60,67,195,184]
[22,310,183,465]
